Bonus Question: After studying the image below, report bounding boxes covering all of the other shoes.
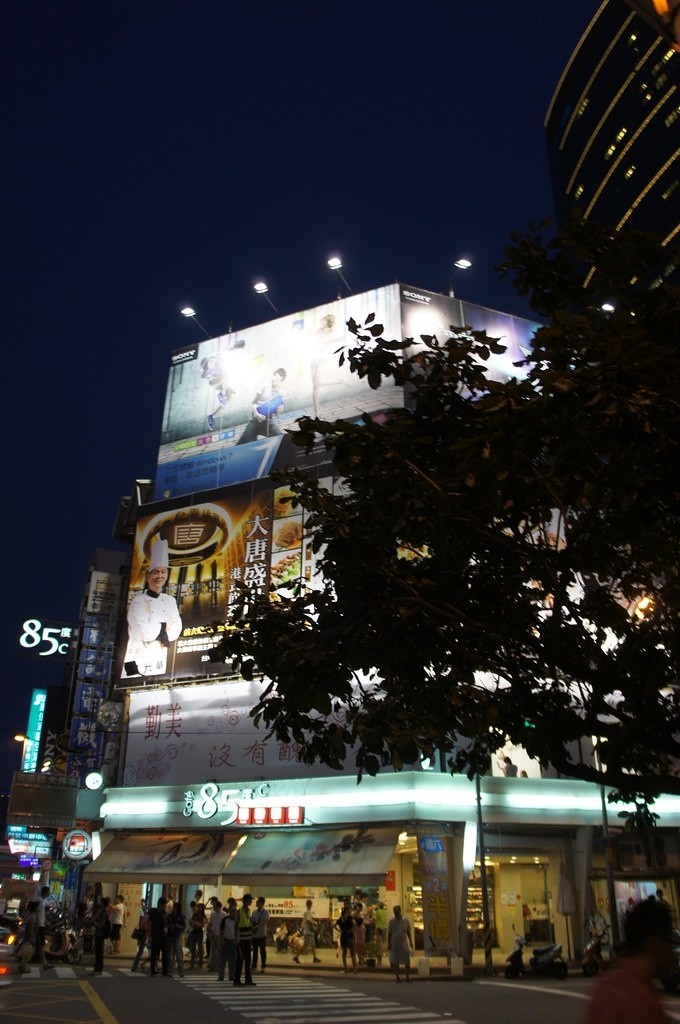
[233,980,244,987]
[313,958,322,963]
[43,962,55,970]
[217,977,224,981]
[89,970,102,976]
[293,956,301,964]
[245,980,256,985]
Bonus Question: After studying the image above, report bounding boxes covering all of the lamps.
[180,306,214,339]
[449,258,472,298]
[327,256,354,295]
[254,281,282,317]
[124,661,149,680]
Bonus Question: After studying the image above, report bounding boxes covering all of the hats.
[243,893,255,902]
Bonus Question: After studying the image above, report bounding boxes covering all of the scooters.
[29,914,83,967]
[505,924,570,983]
[582,920,611,978]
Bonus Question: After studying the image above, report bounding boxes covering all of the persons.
[649,889,669,906]
[216,903,237,981]
[78,887,96,955]
[234,893,257,986]
[274,919,289,952]
[130,894,186,977]
[333,906,358,973]
[585,899,680,1024]
[17,900,41,973]
[9,886,56,970]
[292,900,321,964]
[86,896,110,975]
[251,896,269,974]
[186,889,236,970]
[342,890,388,967]
[287,919,311,952]
[108,895,127,954]
[387,905,414,984]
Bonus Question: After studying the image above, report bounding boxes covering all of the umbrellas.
[556,861,577,961]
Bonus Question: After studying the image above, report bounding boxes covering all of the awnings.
[81,830,244,886]
[221,829,400,886]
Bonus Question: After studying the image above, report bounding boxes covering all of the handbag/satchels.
[16,942,36,961]
[208,936,228,958]
[131,924,143,940]
[99,909,111,939]
[144,935,151,949]
